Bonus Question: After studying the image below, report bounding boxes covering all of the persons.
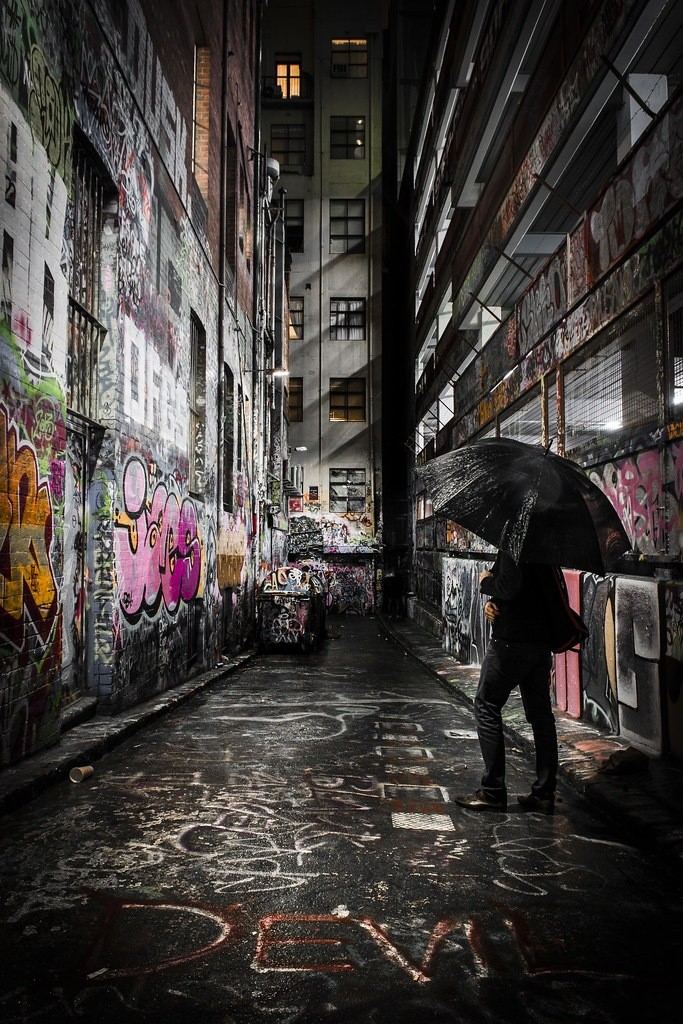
[288,553,310,572]
[456,550,562,814]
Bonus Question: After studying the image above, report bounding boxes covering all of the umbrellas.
[415,439,633,578]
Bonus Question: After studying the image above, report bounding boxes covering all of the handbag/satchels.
[550,565,590,653]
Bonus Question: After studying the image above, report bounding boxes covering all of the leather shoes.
[517,794,554,816]
[455,788,507,813]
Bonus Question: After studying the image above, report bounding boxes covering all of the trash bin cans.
[258,566,329,654]
